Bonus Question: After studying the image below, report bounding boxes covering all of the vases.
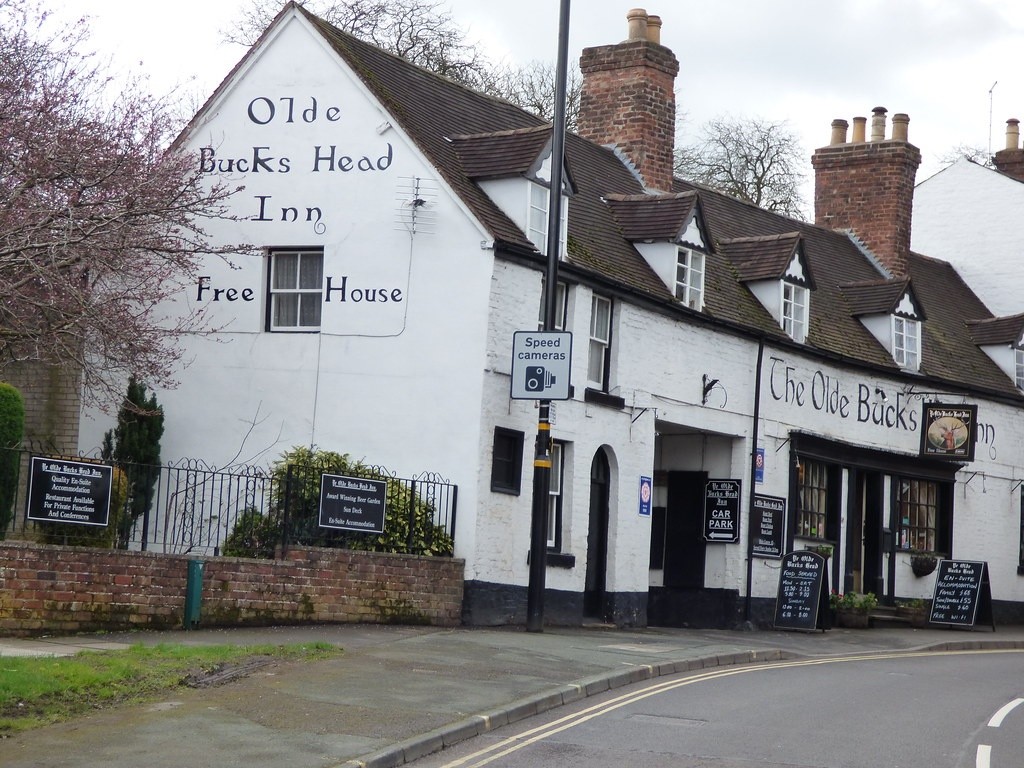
[831,608,872,628]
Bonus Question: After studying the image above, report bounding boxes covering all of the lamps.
[875,387,888,402]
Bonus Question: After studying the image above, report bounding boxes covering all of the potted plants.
[895,593,933,625]
[910,549,938,578]
[808,545,835,562]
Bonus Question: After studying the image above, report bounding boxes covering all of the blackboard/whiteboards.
[928,559,994,626]
[773,550,830,632]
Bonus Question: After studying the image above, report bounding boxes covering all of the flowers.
[828,589,877,609]
